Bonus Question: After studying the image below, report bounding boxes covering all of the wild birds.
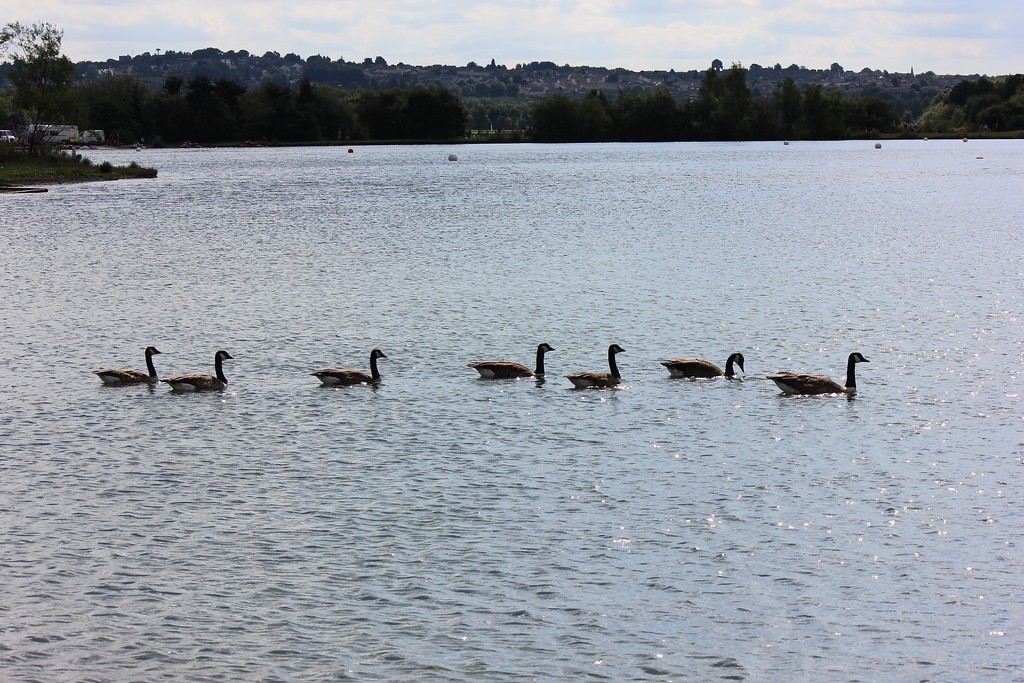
[562,344,626,387]
[659,352,744,377]
[766,352,870,394]
[94,347,163,385]
[467,343,556,378]
[309,349,387,384]
[159,351,234,391]
